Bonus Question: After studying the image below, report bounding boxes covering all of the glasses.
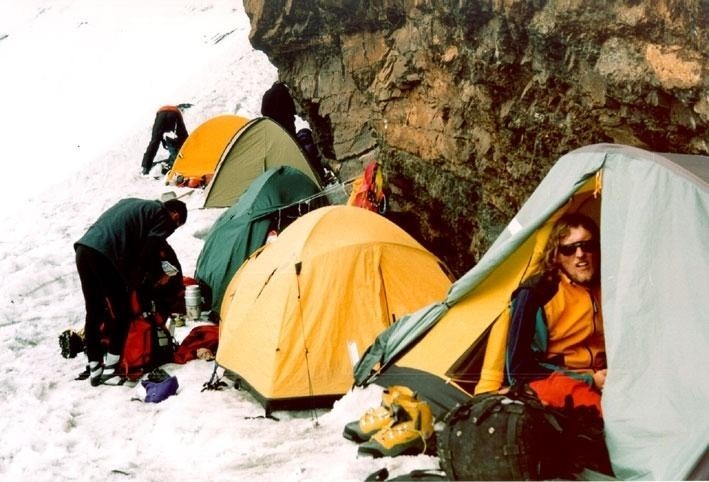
[557,241,598,256]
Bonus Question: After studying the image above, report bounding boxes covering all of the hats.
[187,176,204,188]
[139,375,180,404]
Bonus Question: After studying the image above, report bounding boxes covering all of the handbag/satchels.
[437,390,570,482]
[98,314,178,384]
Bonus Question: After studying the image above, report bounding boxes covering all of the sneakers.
[88,352,138,389]
[342,384,440,461]
[141,167,151,176]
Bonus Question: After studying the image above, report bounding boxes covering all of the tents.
[208,203,454,417]
[194,165,332,320]
[198,117,322,209]
[167,114,250,181]
[367,142,709,480]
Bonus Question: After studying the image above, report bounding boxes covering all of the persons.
[75,198,187,382]
[506,211,607,417]
[262,81,298,136]
[142,106,188,174]
